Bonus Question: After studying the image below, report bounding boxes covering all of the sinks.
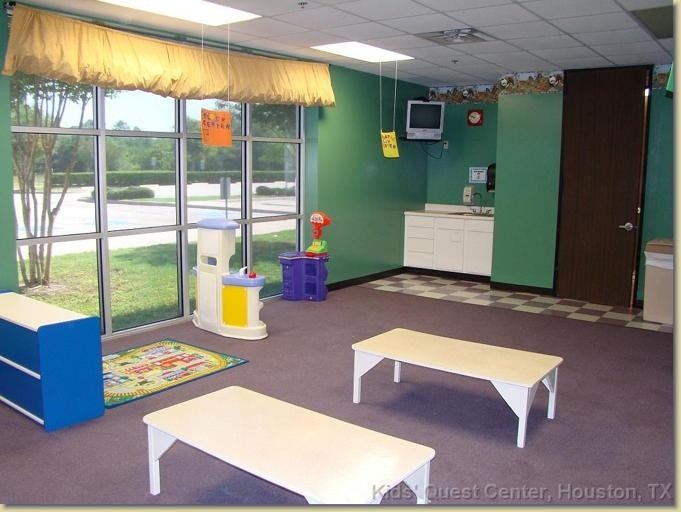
[448,211,493,216]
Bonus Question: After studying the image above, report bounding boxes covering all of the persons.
[277,250,329,301]
[305,211,331,257]
[191,217,269,340]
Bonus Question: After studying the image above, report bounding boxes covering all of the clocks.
[466,108,484,126]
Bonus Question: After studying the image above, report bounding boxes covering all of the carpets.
[101,335,252,409]
[0,288,677,507]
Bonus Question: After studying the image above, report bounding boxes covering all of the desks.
[349,327,568,451]
[138,382,438,506]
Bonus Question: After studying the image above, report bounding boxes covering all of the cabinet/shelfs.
[403,212,434,269]
[0,287,108,435]
[639,237,675,325]
[434,217,494,276]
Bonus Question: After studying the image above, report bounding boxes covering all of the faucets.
[472,192,483,213]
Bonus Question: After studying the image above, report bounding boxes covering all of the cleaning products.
[463,183,472,206]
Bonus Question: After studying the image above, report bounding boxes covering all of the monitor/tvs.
[405,100,445,140]
